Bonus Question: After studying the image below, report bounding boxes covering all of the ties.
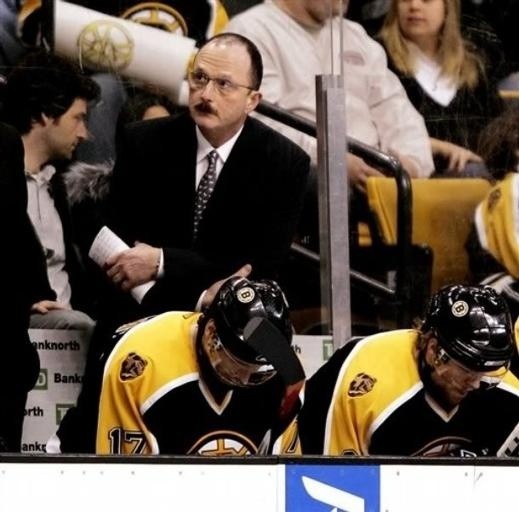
[192,150,221,240]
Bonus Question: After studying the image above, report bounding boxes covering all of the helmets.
[203,274,295,366]
[426,282,519,371]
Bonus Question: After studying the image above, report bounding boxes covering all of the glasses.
[188,70,255,96]
